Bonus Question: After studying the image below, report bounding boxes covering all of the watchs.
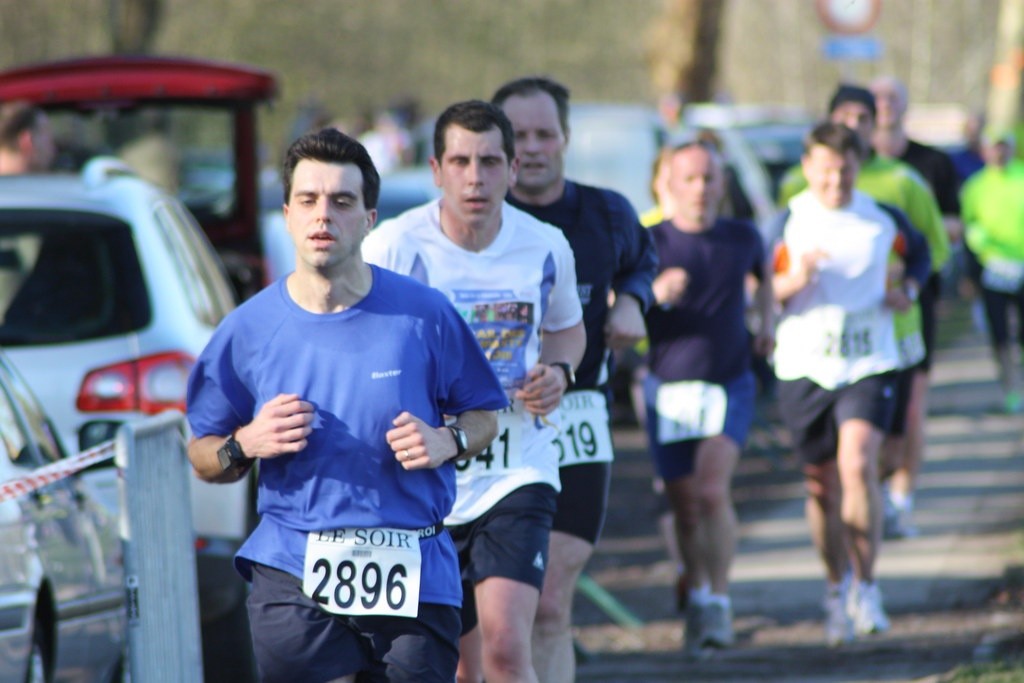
[447,425,469,460]
[217,426,257,478]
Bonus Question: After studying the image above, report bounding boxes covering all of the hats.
[980,123,1013,144]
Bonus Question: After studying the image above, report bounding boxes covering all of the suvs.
[0,152,263,629]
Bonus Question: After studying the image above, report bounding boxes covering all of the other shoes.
[1004,390,1020,413]
[824,585,857,649]
[683,613,712,662]
[700,593,734,647]
[846,581,888,638]
[878,481,915,539]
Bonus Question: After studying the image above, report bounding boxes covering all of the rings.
[404,449,410,461]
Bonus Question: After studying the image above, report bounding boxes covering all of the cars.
[0,349,130,682]
[0,51,807,432]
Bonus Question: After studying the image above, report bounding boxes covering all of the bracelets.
[547,361,575,393]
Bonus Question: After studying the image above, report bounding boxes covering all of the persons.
[486,75,662,683]
[181,127,511,683]
[638,73,1023,662]
[0,99,118,321]
[313,95,587,683]
